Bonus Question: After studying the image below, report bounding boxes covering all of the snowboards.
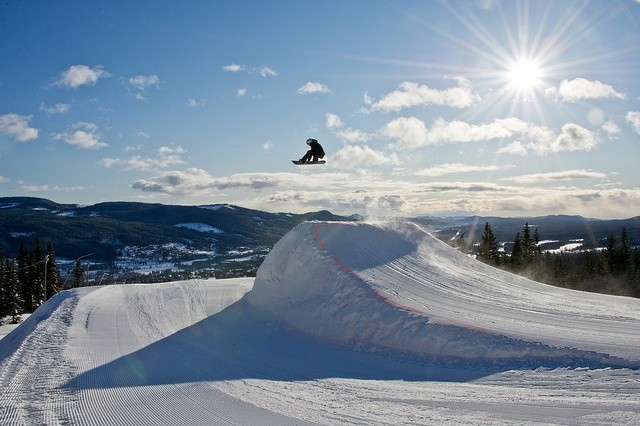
[292,160,325,165]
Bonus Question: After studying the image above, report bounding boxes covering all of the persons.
[299,139,325,164]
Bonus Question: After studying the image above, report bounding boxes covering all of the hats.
[306,138,312,143]
